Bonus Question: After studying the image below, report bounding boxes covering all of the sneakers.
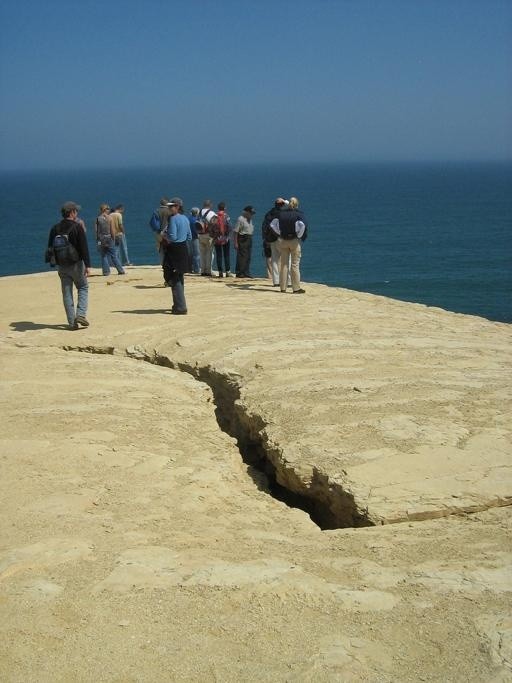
[201,273,253,278]
[274,284,305,293]
[69,317,89,331]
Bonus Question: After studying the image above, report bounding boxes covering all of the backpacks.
[194,208,212,234]
[51,223,79,265]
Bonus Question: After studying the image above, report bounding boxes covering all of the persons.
[94,204,128,276]
[160,197,193,315]
[48,201,91,330]
[74,217,87,233]
[150,196,308,293]
[109,203,133,267]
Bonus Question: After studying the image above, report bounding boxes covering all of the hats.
[160,197,182,208]
[61,201,82,211]
[244,207,256,214]
[276,197,289,205]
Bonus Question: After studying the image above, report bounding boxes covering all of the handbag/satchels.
[150,208,161,231]
[208,215,220,238]
